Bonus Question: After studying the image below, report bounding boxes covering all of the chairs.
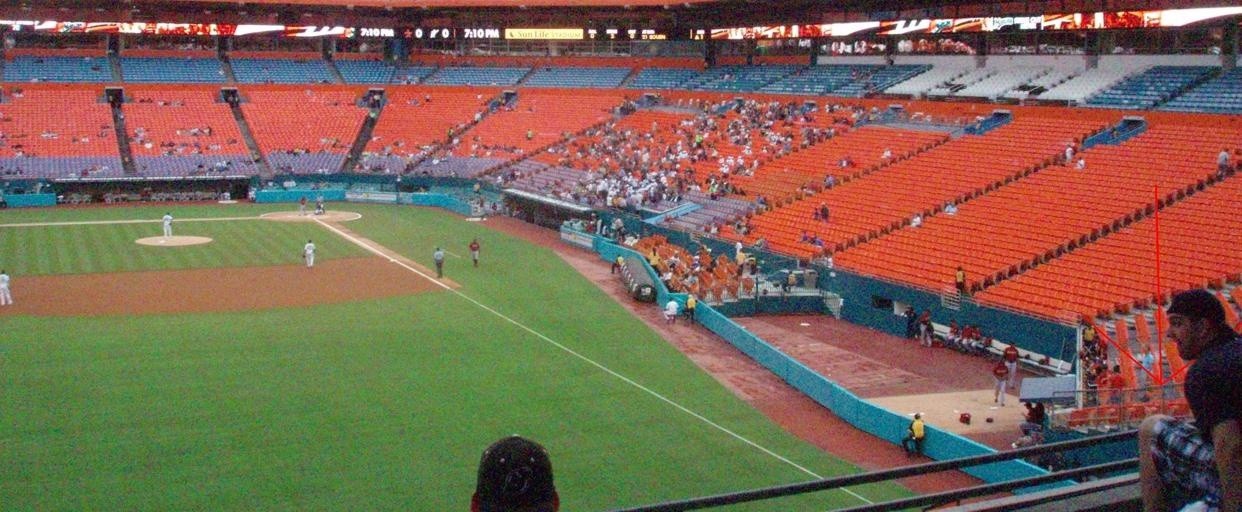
[1,50,1241,322]
[1056,284,1242,427]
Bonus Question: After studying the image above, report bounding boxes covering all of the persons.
[992,340,1019,407]
[1013,402,1049,467]
[902,413,925,458]
[3,59,1242,344]
[1139,287,1241,511]
[471,436,560,511]
[1080,336,1155,406]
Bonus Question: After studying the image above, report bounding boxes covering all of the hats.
[1167,289,1238,337]
[476,436,552,508]
